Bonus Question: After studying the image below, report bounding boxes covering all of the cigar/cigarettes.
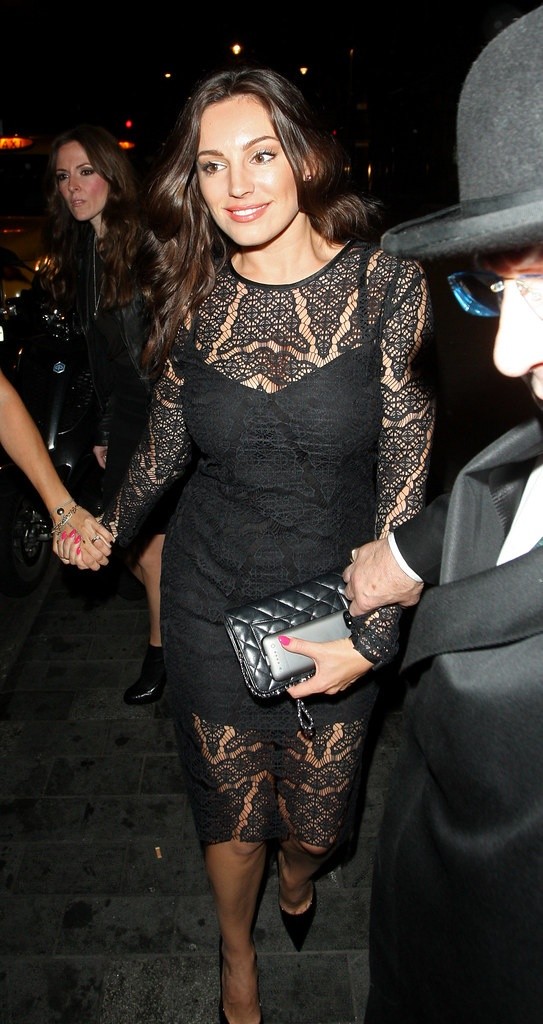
[155,847,162,858]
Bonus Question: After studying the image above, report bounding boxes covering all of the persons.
[0,70,433,1024]
[342,6,543,1024]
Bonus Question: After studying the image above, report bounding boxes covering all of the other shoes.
[277,858,317,952]
[218,932,264,1024]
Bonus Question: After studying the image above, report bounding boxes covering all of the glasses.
[448,268,543,317]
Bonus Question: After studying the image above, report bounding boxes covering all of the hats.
[381,8,543,258]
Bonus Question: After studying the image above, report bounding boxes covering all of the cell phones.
[262,609,357,681]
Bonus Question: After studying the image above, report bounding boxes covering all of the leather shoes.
[123,644,166,705]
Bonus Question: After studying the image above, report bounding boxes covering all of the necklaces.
[93,232,103,318]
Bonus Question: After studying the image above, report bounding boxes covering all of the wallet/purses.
[223,564,357,698]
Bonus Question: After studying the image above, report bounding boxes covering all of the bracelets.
[50,499,86,543]
[52,504,81,533]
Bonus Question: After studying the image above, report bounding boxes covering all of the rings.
[349,548,357,562]
[91,536,101,543]
[104,455,106,458]
